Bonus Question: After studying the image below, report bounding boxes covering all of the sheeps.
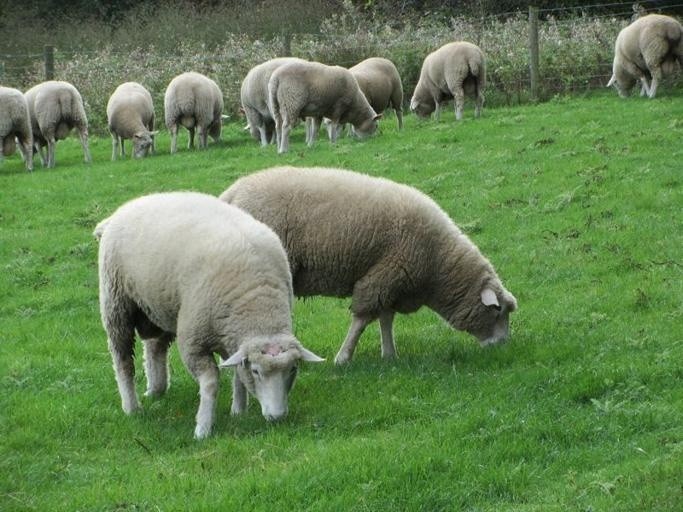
[164,71,224,155]
[216,164,518,367]
[410,41,487,122]
[606,14,683,99]
[240,55,404,154]
[92,188,327,442]
[106,81,155,162]
[15,80,91,168]
[0,86,34,171]
